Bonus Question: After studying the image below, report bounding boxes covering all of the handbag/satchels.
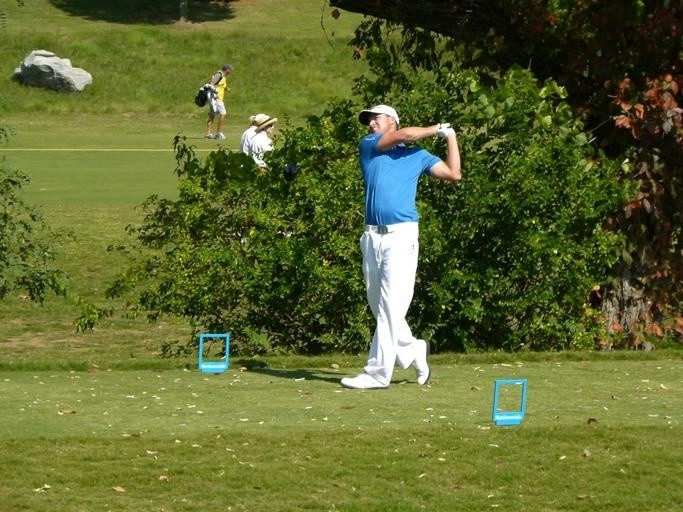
[195,88,208,106]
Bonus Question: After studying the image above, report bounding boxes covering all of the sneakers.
[341,373,388,388]
[205,133,225,139]
[413,339,430,385]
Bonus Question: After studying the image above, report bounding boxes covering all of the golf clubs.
[282,124,454,182]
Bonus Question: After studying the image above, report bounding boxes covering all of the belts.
[365,222,416,233]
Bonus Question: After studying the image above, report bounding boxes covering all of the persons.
[239,114,257,155]
[247,113,279,174]
[338,103,462,390]
[204,63,232,138]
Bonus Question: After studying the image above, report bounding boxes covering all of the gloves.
[438,123,454,137]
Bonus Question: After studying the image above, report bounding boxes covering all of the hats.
[359,105,399,125]
[254,113,277,132]
